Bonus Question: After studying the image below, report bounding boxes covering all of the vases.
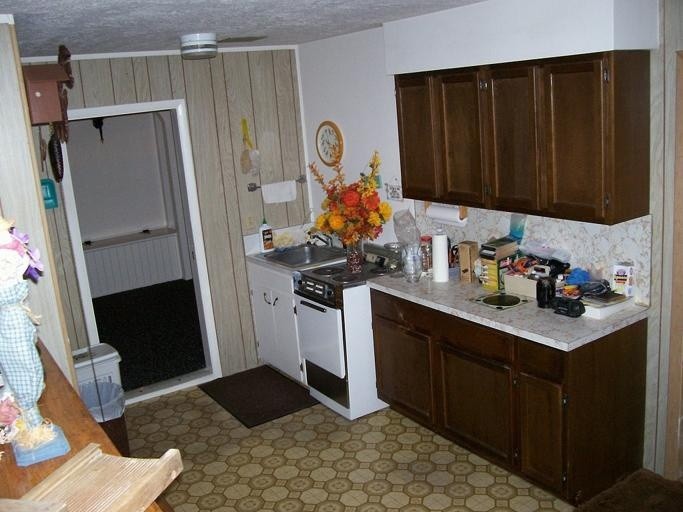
[345,238,362,275]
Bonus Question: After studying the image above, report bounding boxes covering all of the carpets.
[568,468,682,511]
[197,365,321,428]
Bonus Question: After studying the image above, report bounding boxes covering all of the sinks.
[254,245,347,268]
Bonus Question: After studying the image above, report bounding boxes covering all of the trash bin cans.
[72,343,130,457]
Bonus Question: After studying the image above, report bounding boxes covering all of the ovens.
[293,289,346,382]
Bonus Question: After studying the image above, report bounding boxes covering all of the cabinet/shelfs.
[369,288,648,506]
[245,261,304,383]
[393,50,651,226]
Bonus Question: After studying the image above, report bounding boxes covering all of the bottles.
[385,228,450,283]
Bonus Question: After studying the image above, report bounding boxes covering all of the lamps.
[179,33,217,60]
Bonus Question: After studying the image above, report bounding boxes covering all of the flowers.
[307,149,393,245]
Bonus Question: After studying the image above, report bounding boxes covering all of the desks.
[0,337,173,512]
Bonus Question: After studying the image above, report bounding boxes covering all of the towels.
[261,179,297,204]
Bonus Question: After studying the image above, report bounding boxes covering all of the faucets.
[310,234,329,245]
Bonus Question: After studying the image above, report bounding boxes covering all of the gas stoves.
[300,262,387,287]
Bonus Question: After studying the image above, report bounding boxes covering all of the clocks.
[315,121,343,166]
